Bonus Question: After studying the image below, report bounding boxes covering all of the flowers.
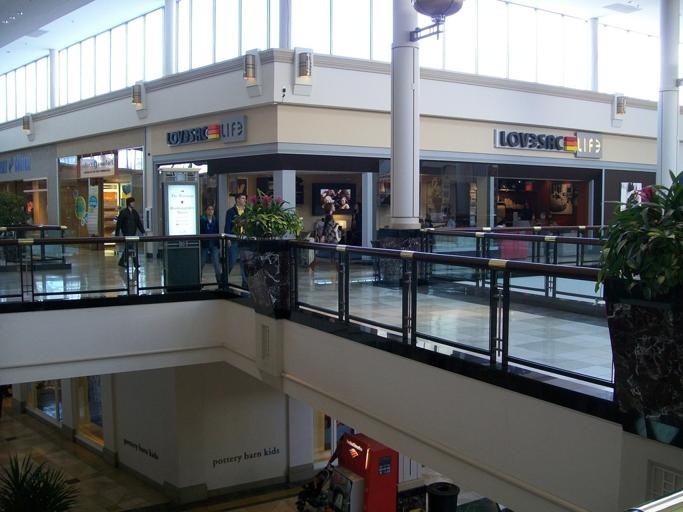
[229,187,304,251]
[594,169,683,301]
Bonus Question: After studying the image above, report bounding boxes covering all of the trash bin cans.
[427,481,460,512]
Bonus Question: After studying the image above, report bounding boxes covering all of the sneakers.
[119,262,141,269]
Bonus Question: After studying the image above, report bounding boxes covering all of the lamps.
[23,113,34,141]
[293,47,313,96]
[132,80,147,118]
[244,49,261,96]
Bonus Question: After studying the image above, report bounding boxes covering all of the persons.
[520,198,535,221]
[219,192,249,296]
[325,202,342,244]
[351,201,361,237]
[320,189,350,211]
[199,204,222,289]
[115,197,146,269]
